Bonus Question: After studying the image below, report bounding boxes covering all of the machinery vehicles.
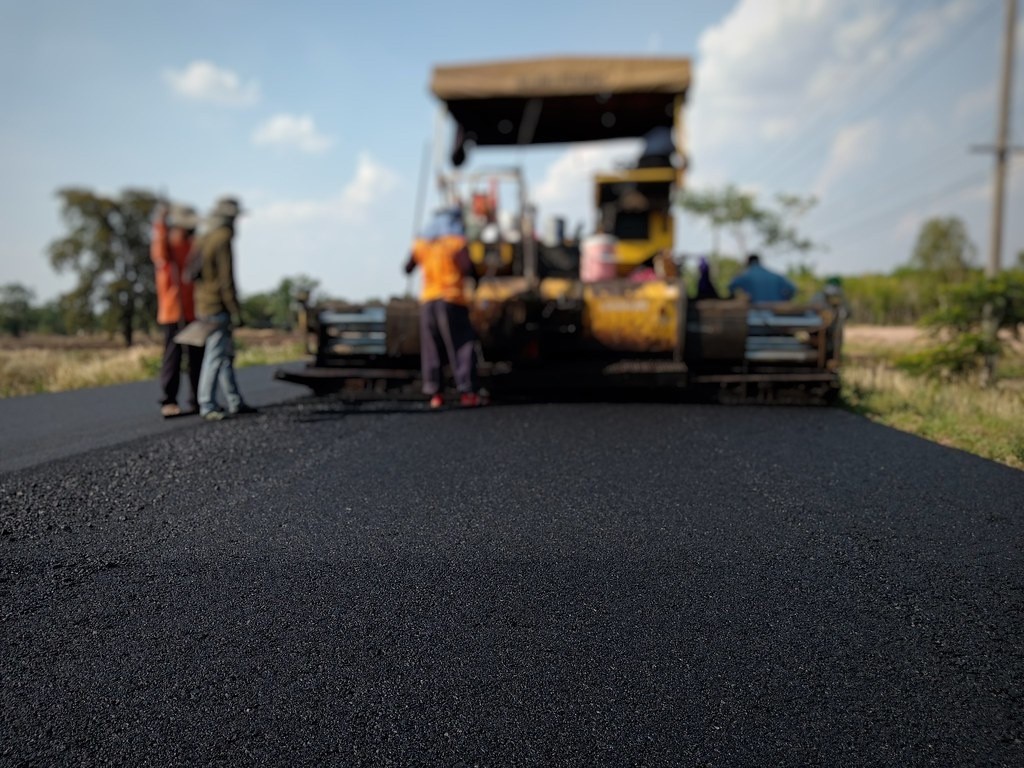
[274,53,846,408]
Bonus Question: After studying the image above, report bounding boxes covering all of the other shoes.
[204,403,227,421]
[233,400,257,413]
[161,403,198,418]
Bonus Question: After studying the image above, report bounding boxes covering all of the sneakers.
[425,392,490,409]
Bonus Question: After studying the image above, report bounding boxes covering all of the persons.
[184,199,257,421]
[400,198,488,408]
[695,256,721,299]
[804,274,852,373]
[635,125,680,210]
[728,254,797,306]
[499,213,548,276]
[547,219,579,277]
[296,290,320,353]
[148,195,210,418]
[630,255,664,283]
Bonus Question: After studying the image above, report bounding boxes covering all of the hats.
[210,198,239,217]
[164,207,196,230]
